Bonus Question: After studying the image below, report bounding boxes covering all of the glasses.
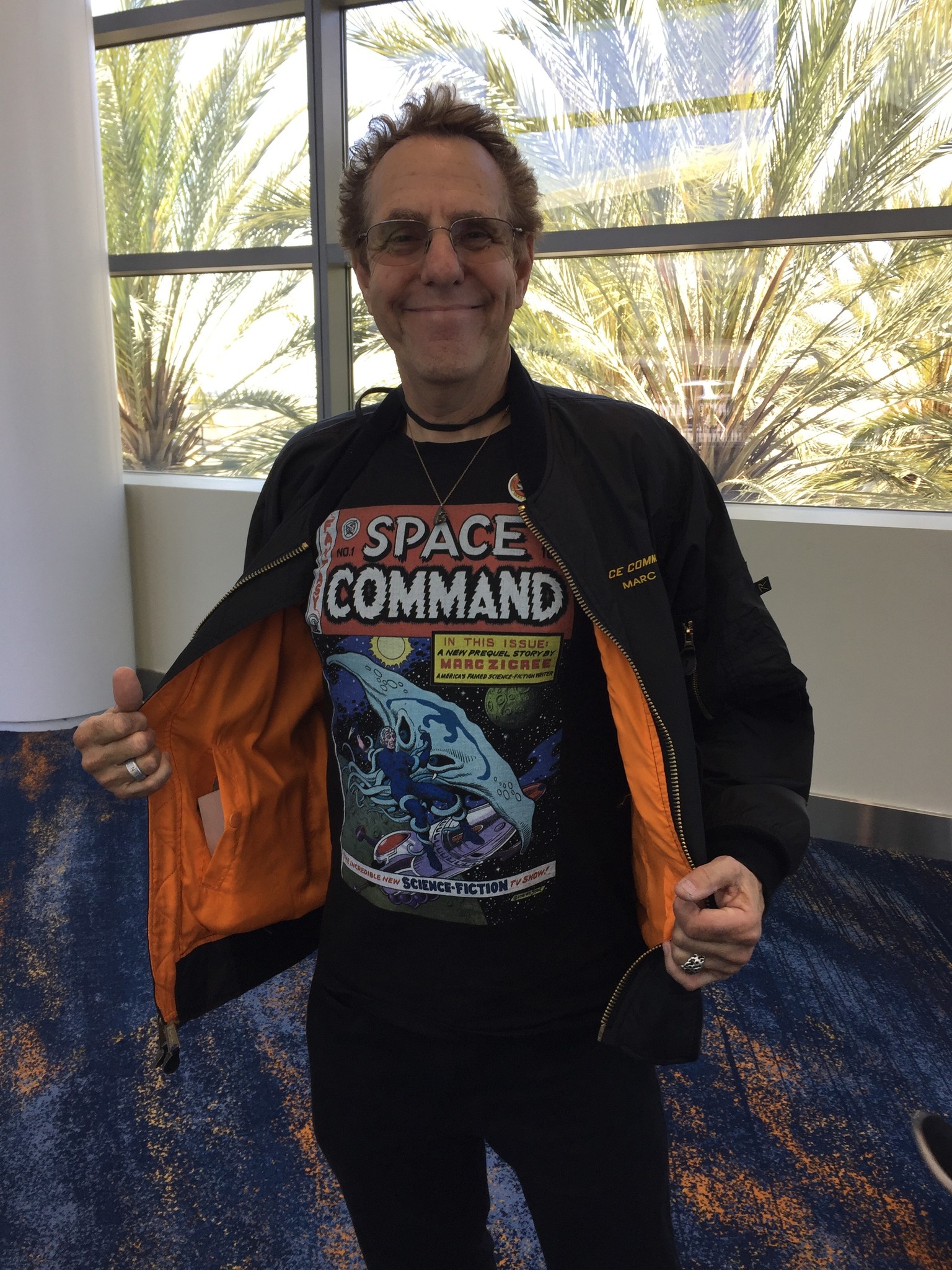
[354,217,528,267]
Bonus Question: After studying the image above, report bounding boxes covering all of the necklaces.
[399,383,508,432]
[406,407,509,525]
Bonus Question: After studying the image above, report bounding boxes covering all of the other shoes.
[911,1110,952,1191]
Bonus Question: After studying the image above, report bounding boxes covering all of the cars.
[671,379,741,423]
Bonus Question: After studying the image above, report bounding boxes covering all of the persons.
[70,86,816,1270]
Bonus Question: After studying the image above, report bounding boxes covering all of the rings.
[680,954,705,975]
[125,759,146,781]
